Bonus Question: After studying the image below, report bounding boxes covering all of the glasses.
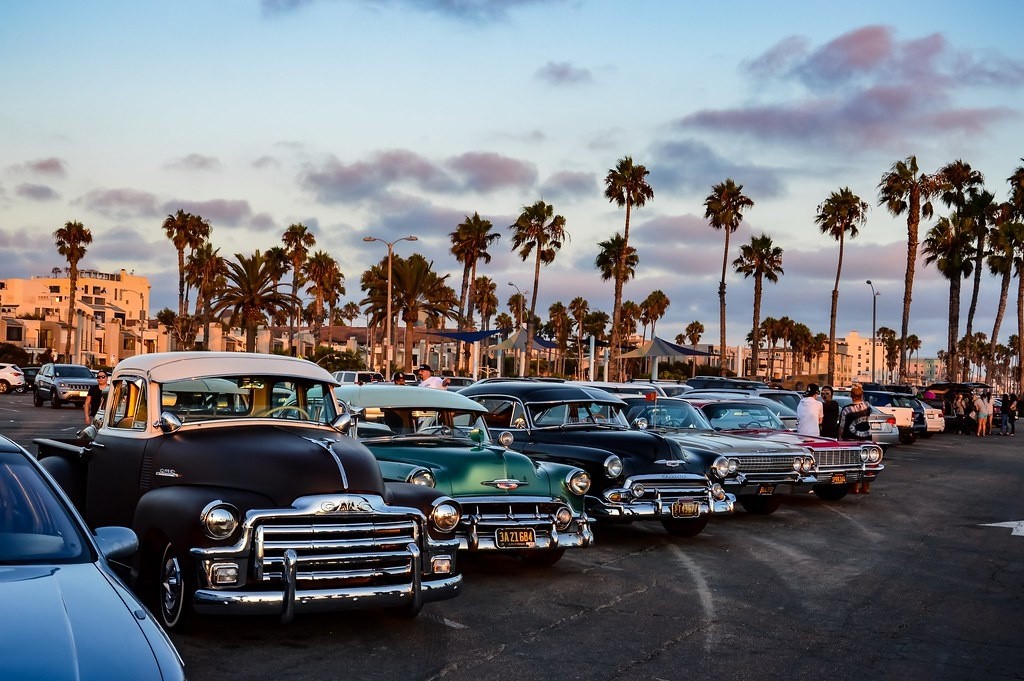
[97,375,107,378]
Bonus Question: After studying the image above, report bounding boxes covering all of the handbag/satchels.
[1010,410,1019,420]
[969,411,978,423]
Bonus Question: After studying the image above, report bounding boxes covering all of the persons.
[839,383,874,494]
[39,349,55,365]
[819,386,840,439]
[794,381,804,391]
[417,363,451,391]
[951,391,1024,437]
[796,383,824,436]
[393,371,406,385]
[84,370,109,426]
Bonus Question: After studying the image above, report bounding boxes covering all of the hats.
[803,383,819,396]
[394,372,405,380]
[419,363,434,371]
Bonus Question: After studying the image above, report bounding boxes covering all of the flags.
[645,392,657,401]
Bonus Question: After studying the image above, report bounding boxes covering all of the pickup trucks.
[331,370,395,386]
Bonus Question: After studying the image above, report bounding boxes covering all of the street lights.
[120,288,144,354]
[866,280,881,383]
[508,282,530,326]
[363,236,418,382]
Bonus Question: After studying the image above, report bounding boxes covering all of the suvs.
[33,362,99,409]
[32,350,464,635]
[0,363,26,394]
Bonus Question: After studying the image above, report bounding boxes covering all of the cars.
[274,384,597,568]
[817,395,899,455]
[390,371,476,392]
[455,382,736,537]
[566,376,803,431]
[91,378,293,423]
[15,367,41,393]
[819,382,1019,445]
[684,399,885,501]
[90,370,112,385]
[0,434,188,681]
[609,393,820,515]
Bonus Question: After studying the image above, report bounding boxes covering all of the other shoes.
[848,487,870,495]
